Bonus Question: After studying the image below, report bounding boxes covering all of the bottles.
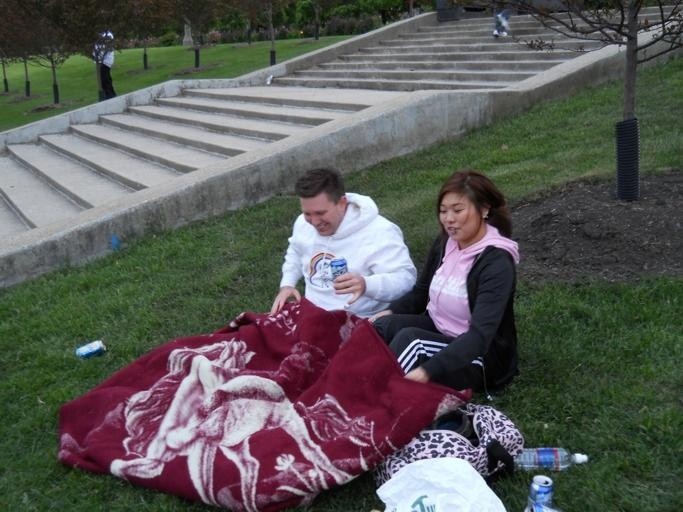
[512,446,590,473]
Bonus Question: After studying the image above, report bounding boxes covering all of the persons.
[368,170,520,390]
[492,5,509,37]
[270,167,418,318]
[92,24,116,100]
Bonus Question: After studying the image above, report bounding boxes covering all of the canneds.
[329,258,348,281]
[524,502,558,512]
[76,341,108,359]
[528,475,553,507]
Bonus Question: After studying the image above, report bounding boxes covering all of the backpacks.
[374,402,527,490]
